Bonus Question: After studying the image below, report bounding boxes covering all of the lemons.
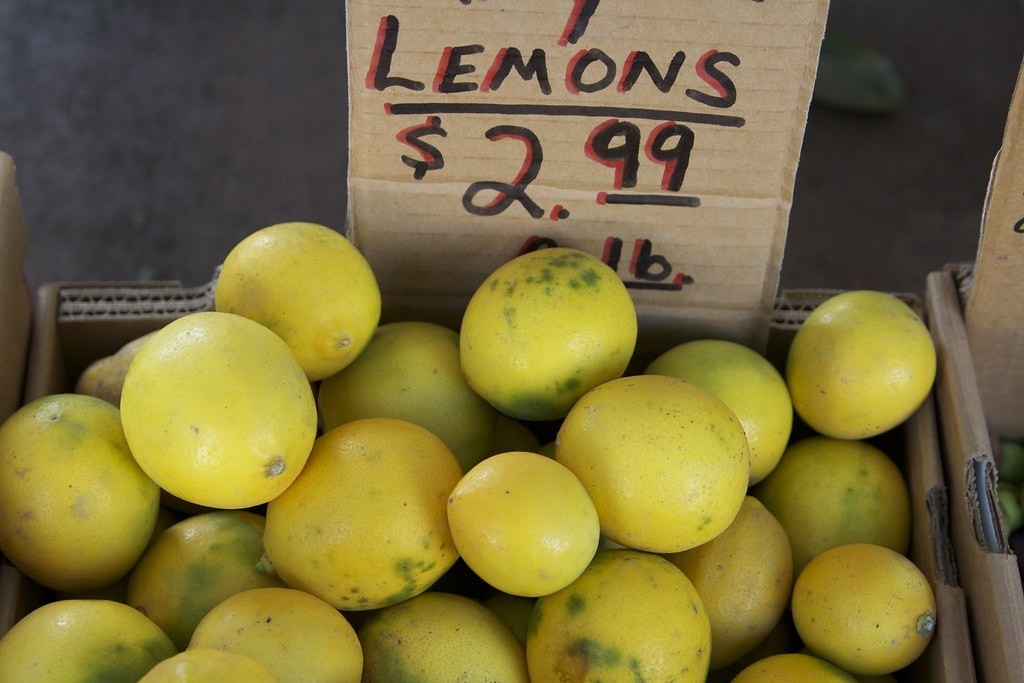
[0,321,935,683]
[786,290,935,441]
[214,221,381,381]
[458,248,639,418]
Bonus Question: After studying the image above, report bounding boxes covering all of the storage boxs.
[0,268,1024,683]
